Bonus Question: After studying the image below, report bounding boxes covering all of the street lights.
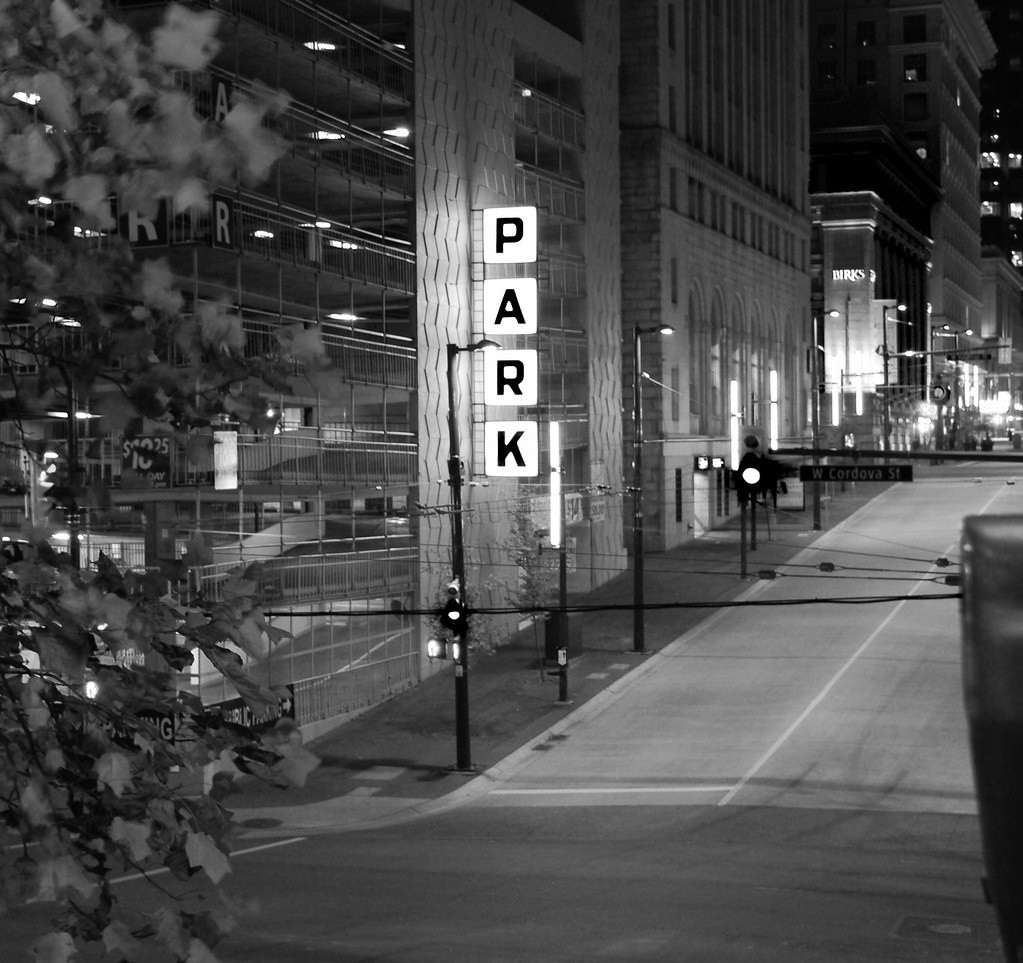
[812,310,839,531]
[883,303,904,463]
[632,321,675,654]
[444,340,499,769]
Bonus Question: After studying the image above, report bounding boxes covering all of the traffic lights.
[444,600,463,635]
[739,457,763,492]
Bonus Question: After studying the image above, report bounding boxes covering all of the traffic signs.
[799,465,912,481]
